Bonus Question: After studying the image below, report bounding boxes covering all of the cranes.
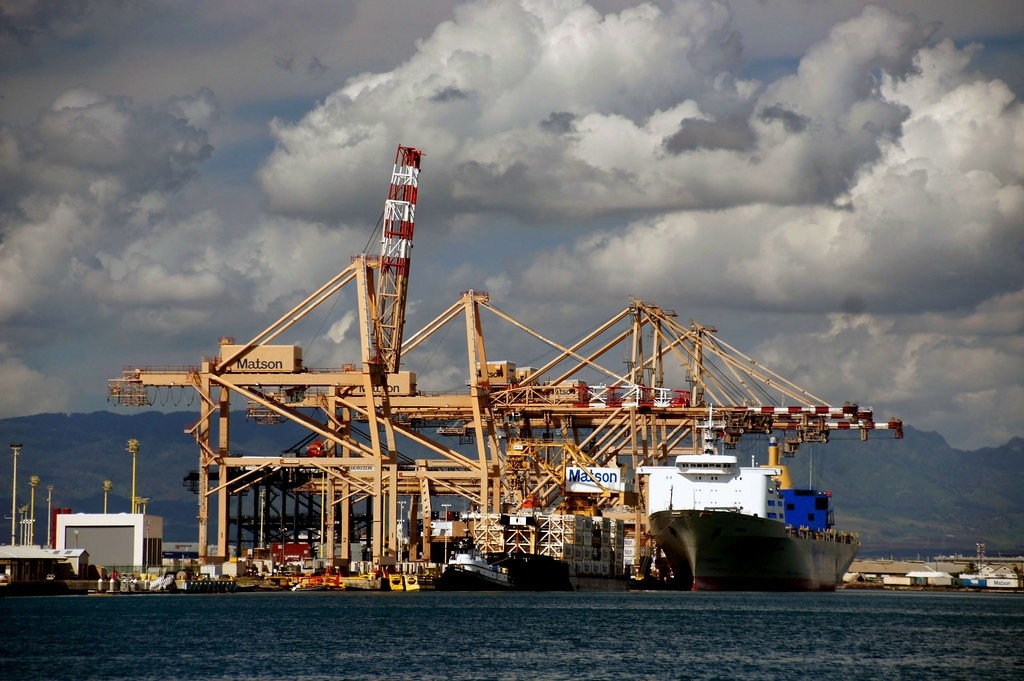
[108,144,906,564]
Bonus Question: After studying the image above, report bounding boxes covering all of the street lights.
[10,444,22,546]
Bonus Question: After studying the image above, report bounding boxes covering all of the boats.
[635,400,862,594]
[438,528,519,592]
